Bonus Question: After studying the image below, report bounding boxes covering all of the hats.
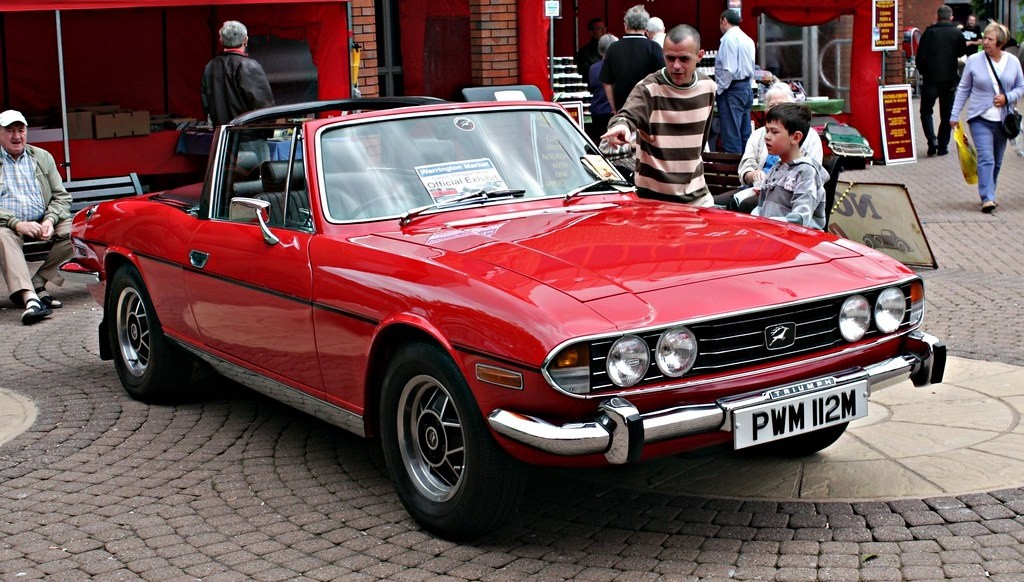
[0,109,28,127]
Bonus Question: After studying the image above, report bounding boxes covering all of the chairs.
[405,138,465,207]
[253,159,368,233]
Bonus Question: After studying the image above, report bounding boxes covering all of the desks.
[750,98,846,115]
[26,130,198,181]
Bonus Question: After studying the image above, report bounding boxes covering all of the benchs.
[700,151,845,233]
[187,139,397,220]
[22,173,143,262]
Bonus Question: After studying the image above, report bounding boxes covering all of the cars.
[60,98,949,538]
[903,27,923,57]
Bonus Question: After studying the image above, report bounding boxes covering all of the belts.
[731,78,751,82]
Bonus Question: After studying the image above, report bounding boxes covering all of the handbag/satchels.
[1002,113,1022,140]
[954,116,978,186]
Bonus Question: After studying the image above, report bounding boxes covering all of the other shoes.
[927,139,937,156]
[980,201,996,213]
[938,149,948,156]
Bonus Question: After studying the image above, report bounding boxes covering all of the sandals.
[34,286,62,309]
[21,298,53,325]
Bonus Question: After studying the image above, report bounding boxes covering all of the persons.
[714,9,756,154]
[573,5,667,183]
[728,82,823,213]
[956,13,983,76]
[750,103,830,232]
[599,25,718,209]
[949,23,1024,213]
[0,110,73,324]
[915,5,965,156]
[201,20,276,176]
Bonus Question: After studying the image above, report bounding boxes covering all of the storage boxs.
[26,106,150,143]
